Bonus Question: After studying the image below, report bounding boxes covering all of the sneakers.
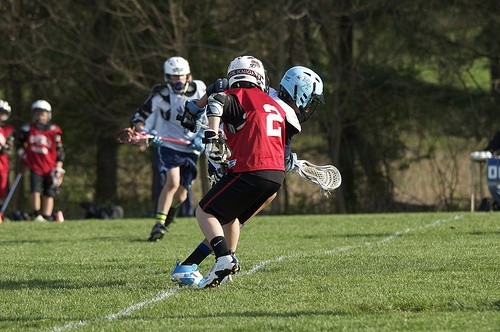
[171,259,203,287]
[148,222,166,242]
[164,207,178,226]
[198,249,242,289]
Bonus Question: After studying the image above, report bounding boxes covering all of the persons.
[171,66,323,286]
[196,56,286,288]
[17,100,65,222]
[0,99,15,223]
[131,56,207,241]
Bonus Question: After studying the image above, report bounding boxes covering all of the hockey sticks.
[114,127,191,146]
[217,150,342,193]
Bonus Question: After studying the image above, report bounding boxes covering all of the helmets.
[279,65,326,125]
[164,56,192,94]
[227,56,269,95]
[32,100,51,112]
[0,100,11,113]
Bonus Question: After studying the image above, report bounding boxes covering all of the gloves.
[201,129,236,174]
[140,129,163,145]
[188,126,209,151]
[206,78,229,97]
[285,153,297,173]
